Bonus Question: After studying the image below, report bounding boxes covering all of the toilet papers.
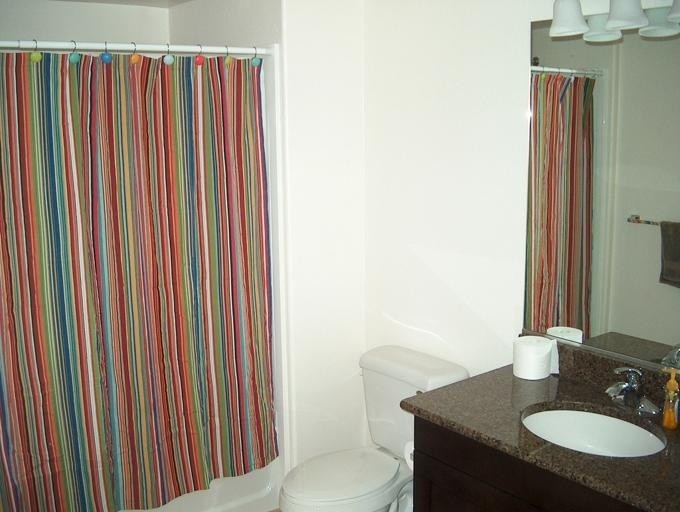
[403,440,414,472]
[512,335,560,381]
[547,326,585,348]
[510,375,559,413]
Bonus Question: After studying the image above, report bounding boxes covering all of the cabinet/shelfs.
[412,418,644,512]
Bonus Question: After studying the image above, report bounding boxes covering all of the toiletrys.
[661,366,679,431]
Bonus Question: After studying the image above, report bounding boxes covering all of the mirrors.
[521,9,680,372]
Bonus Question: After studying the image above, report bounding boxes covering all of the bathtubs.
[0,413,285,512]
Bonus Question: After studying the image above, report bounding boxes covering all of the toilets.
[279,341,469,511]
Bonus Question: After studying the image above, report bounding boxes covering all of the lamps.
[546,0,680,38]
[583,10,678,43]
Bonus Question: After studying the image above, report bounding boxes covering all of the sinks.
[518,399,667,458]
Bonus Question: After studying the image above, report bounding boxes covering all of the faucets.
[660,342,679,369]
[607,367,643,409]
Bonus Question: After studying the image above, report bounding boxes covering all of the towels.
[657,221,680,287]
